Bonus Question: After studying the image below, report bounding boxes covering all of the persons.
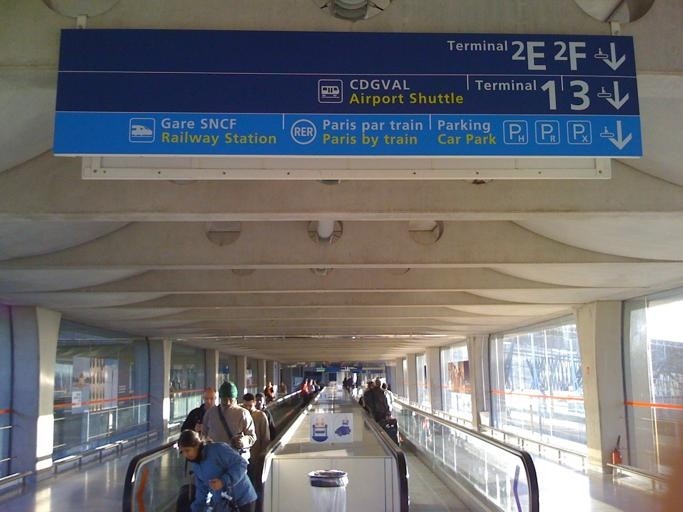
[179,373,395,512]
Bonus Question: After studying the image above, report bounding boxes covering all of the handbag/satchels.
[228,432,252,460]
[209,471,239,511]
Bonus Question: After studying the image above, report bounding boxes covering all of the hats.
[177,429,199,447]
[200,387,215,398]
[217,380,240,399]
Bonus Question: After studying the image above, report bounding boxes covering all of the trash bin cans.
[307,470,348,512]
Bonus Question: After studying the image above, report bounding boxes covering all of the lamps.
[314,220,334,240]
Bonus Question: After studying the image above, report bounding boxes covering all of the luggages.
[176,482,197,512]
[377,417,400,449]
